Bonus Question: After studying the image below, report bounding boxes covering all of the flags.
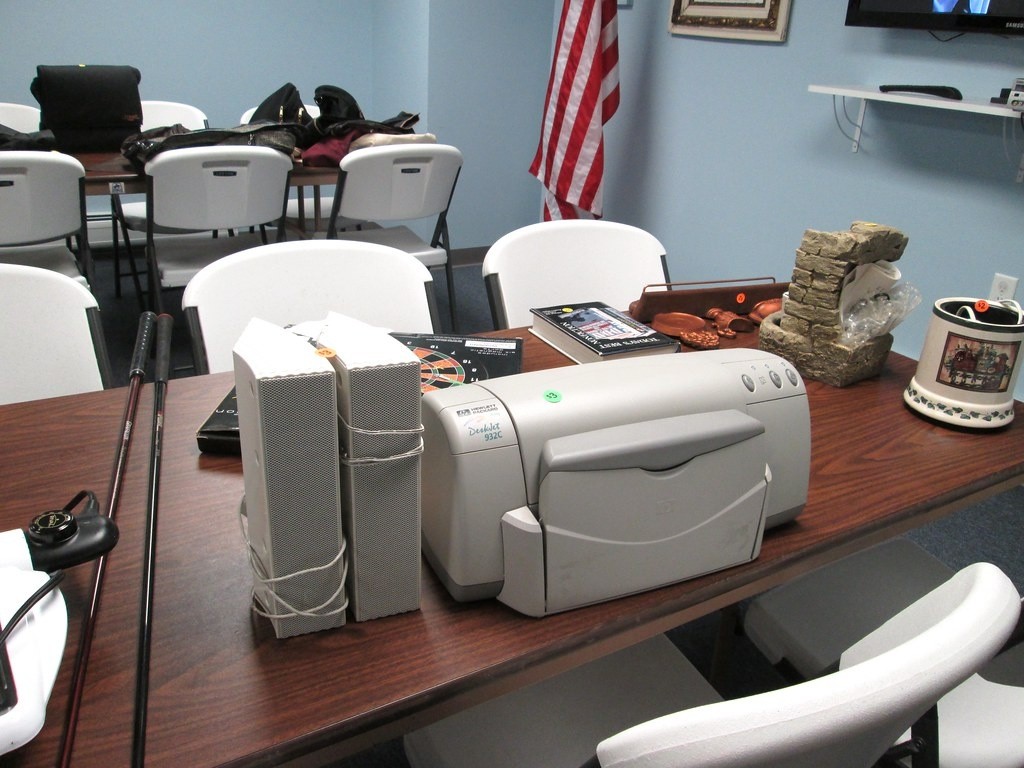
[529,0,622,223]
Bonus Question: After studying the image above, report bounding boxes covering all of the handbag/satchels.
[29,63,309,174]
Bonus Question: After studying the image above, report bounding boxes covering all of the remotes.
[878,84,963,101]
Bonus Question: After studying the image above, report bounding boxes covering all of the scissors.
[850,292,890,315]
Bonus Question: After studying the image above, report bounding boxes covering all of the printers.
[421,348,810,618]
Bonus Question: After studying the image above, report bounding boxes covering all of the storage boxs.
[195,332,521,461]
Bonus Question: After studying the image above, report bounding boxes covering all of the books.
[528,302,682,366]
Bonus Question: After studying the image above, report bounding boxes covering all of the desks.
[0,311,1024,768]
[65,150,342,242]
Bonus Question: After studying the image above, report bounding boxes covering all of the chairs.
[0,98,1024,768]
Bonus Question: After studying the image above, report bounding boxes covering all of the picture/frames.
[668,0,792,43]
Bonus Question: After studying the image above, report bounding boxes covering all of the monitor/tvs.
[843,0,1024,37]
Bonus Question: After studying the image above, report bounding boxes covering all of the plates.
[0,561,68,760]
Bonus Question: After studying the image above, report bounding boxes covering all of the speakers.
[233,315,423,641]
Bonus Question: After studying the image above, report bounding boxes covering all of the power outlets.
[988,272,1019,306]
